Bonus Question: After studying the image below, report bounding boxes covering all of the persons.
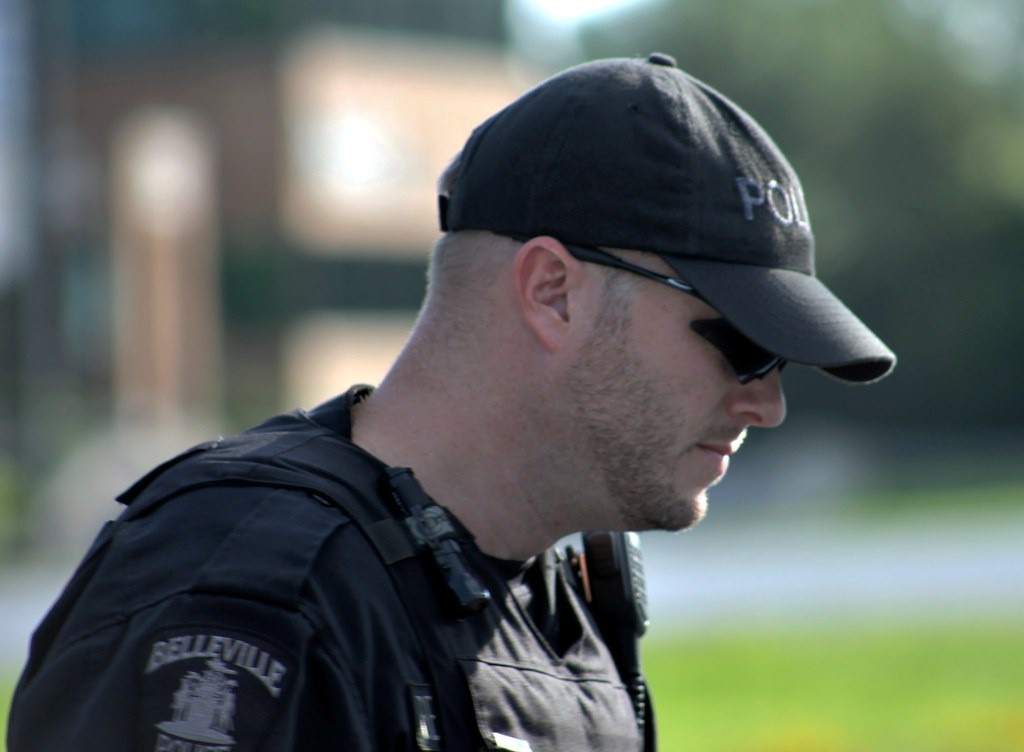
[6,52,898,752]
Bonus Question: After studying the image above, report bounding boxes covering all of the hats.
[437,52,897,385]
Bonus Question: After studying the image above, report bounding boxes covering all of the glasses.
[562,242,790,384]
